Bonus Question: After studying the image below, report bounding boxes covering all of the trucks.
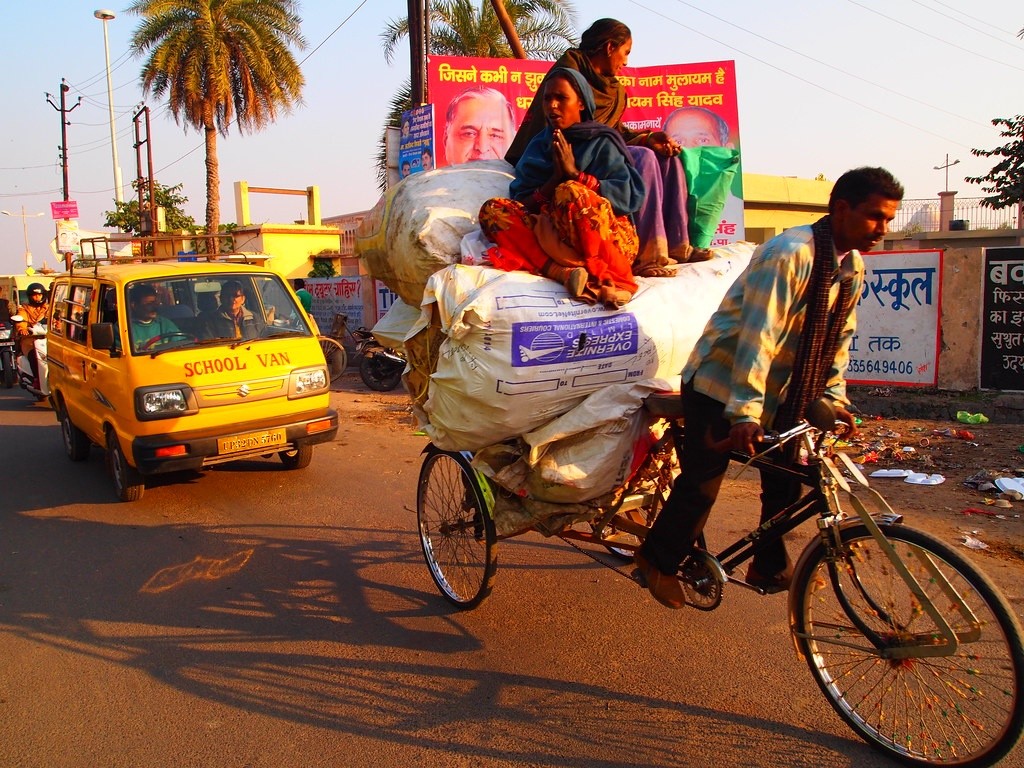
[0,272,64,311]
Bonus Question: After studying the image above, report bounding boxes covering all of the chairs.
[193,292,219,339]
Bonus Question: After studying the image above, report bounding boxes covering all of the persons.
[401,160,410,179]
[112,283,189,353]
[198,280,267,343]
[411,159,420,167]
[14,283,64,390]
[77,288,118,343]
[59,233,70,247]
[442,85,517,168]
[633,166,906,611]
[503,18,715,277]
[420,147,434,171]
[288,278,314,335]
[0,286,19,384]
[401,117,413,139]
[661,105,735,150]
[477,67,647,310]
[70,232,79,246]
[181,289,221,341]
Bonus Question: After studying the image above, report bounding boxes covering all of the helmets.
[26,283,48,304]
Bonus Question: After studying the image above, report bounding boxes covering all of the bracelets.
[576,170,601,193]
[532,187,549,204]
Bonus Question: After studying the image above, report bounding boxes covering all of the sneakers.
[572,265,588,299]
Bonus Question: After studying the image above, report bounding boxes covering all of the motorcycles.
[0,321,17,389]
[352,327,407,391]
[10,314,56,410]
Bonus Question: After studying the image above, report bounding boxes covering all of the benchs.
[82,305,196,339]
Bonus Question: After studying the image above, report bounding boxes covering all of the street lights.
[95,8,132,235]
[2,206,48,268]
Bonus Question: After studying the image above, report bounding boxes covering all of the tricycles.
[409,387,1024,768]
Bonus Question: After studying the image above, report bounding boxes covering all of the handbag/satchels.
[677,145,740,250]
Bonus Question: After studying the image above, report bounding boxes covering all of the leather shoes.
[633,549,686,610]
[744,557,826,594]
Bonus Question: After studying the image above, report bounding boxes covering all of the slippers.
[689,247,715,261]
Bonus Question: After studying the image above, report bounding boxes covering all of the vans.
[46,251,341,502]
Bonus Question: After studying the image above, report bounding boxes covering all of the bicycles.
[315,310,372,382]
